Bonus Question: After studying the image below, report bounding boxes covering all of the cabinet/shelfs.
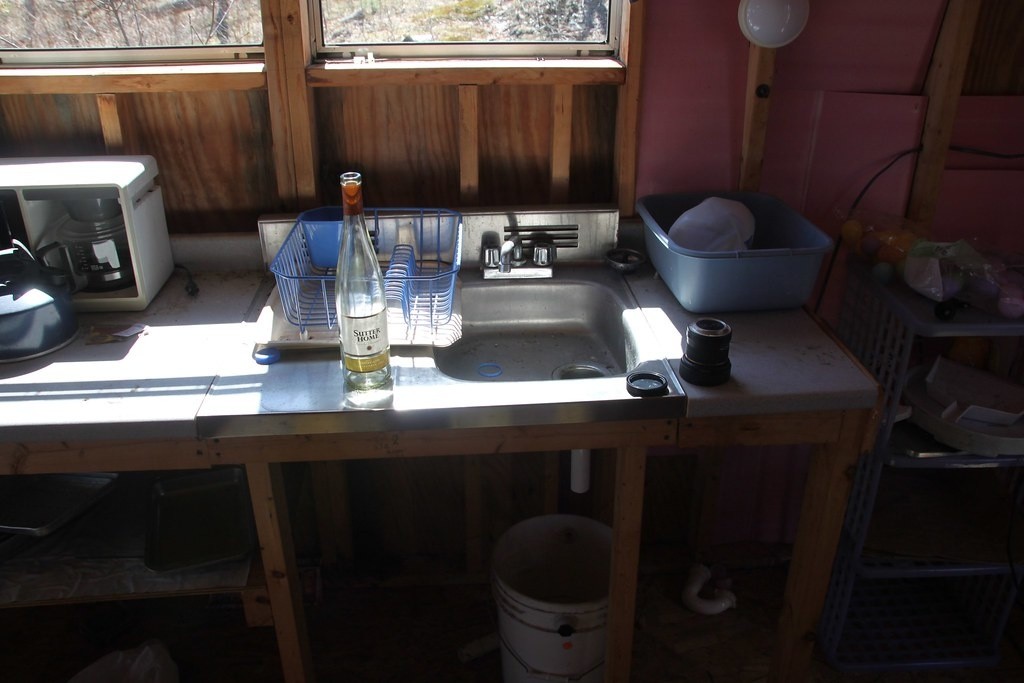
[817,254,1023,676]
[0,435,314,683]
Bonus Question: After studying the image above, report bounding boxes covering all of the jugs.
[37,214,134,298]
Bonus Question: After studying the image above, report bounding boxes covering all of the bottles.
[336,172,394,406]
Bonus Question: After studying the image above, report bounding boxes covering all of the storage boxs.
[636,192,838,315]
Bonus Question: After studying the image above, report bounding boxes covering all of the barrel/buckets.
[490,514,614,683]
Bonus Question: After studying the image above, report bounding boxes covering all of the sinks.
[392,260,691,411]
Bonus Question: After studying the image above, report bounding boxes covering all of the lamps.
[738,0,810,48]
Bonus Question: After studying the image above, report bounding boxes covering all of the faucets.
[477,234,557,280]
